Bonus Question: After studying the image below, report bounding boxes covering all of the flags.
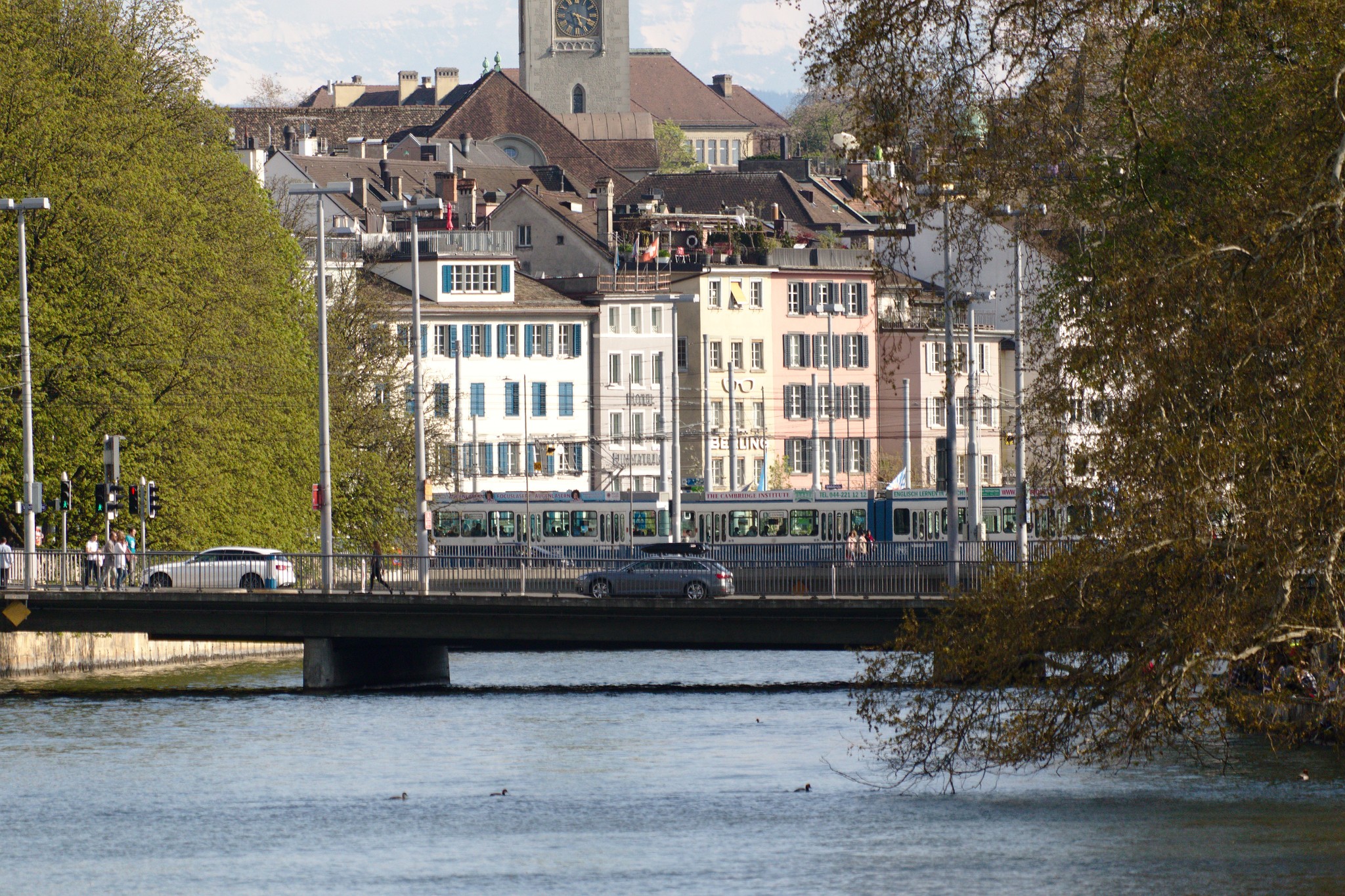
[630,238,639,263]
[614,240,619,274]
[643,237,658,262]
[886,468,906,490]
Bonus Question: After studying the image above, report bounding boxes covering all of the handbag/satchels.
[96,555,103,568]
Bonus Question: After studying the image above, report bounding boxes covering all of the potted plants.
[727,252,741,265]
[701,253,711,264]
[757,230,777,266]
[655,250,670,263]
[619,244,634,261]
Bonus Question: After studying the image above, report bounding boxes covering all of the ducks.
[390,792,408,799]
[793,783,811,793]
[756,718,764,723]
[490,789,508,796]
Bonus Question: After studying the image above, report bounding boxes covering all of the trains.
[421,484,1123,593]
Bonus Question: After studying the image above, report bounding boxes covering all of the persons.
[544,522,598,537]
[0,537,13,587]
[733,527,741,537]
[428,540,439,569]
[746,526,758,537]
[436,520,482,537]
[761,525,770,536]
[85,527,137,591]
[810,523,841,541]
[776,525,786,536]
[682,530,692,557]
[844,524,874,567]
[366,541,393,595]
[633,524,654,536]
[492,523,534,541]
[1004,520,1031,534]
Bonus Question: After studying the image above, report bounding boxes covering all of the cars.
[575,542,735,600]
[140,545,297,590]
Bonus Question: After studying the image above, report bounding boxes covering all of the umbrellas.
[446,203,453,231]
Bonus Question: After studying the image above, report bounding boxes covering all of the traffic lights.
[146,481,162,519]
[94,483,106,514]
[129,485,139,515]
[114,485,125,510]
[60,480,71,511]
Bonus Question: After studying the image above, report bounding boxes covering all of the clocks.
[556,0,599,37]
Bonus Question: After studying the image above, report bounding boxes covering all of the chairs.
[675,247,691,264]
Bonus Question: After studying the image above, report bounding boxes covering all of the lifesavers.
[686,235,698,248]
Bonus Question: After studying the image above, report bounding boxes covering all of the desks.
[692,249,702,263]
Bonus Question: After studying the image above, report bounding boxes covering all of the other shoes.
[390,588,394,594]
[366,592,373,594]
[84,586,91,590]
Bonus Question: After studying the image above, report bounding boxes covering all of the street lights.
[381,196,445,597]
[912,182,963,600]
[287,179,354,598]
[984,203,1049,608]
[0,196,52,593]
